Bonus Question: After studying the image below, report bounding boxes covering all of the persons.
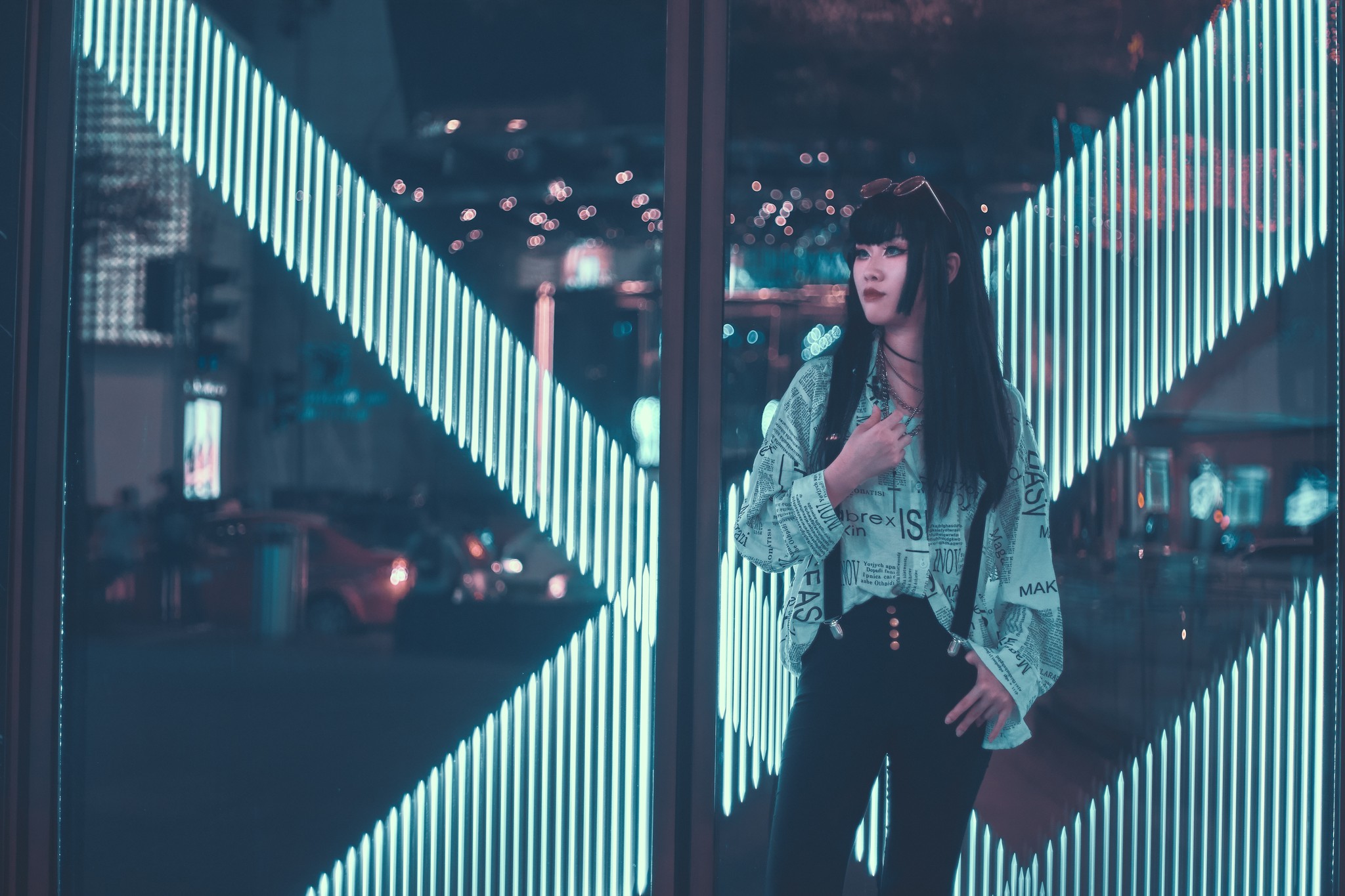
[733,185,1063,896]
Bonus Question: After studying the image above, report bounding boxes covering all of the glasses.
[858,175,951,223]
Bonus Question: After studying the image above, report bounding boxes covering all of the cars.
[100,506,419,642]
[1226,536,1318,579]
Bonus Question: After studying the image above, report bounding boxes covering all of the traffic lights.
[171,251,241,363]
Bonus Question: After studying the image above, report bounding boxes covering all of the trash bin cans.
[249,543,289,647]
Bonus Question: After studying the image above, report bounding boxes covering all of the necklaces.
[876,339,927,441]
